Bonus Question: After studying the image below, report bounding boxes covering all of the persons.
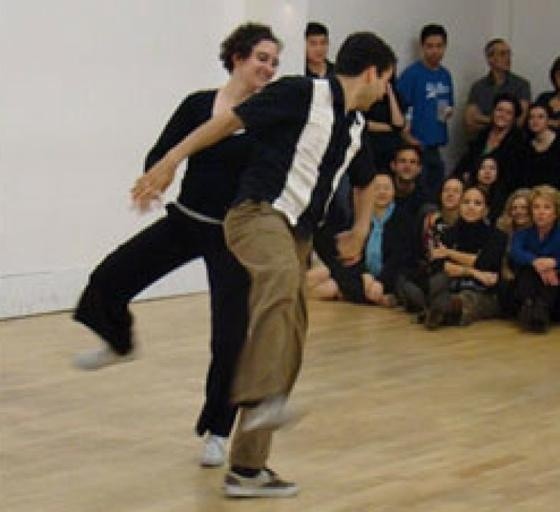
[69,21,282,470]
[307,21,560,332]
[132,31,399,498]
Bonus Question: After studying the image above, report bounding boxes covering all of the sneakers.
[76,342,137,371]
[201,401,300,497]
